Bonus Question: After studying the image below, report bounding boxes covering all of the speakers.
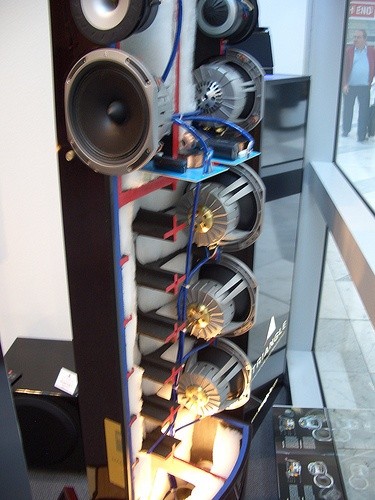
[2,333,81,473]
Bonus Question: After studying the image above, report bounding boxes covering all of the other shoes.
[358,137,368,143]
[341,128,351,137]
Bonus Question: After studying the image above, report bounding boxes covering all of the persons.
[341,28,375,142]
